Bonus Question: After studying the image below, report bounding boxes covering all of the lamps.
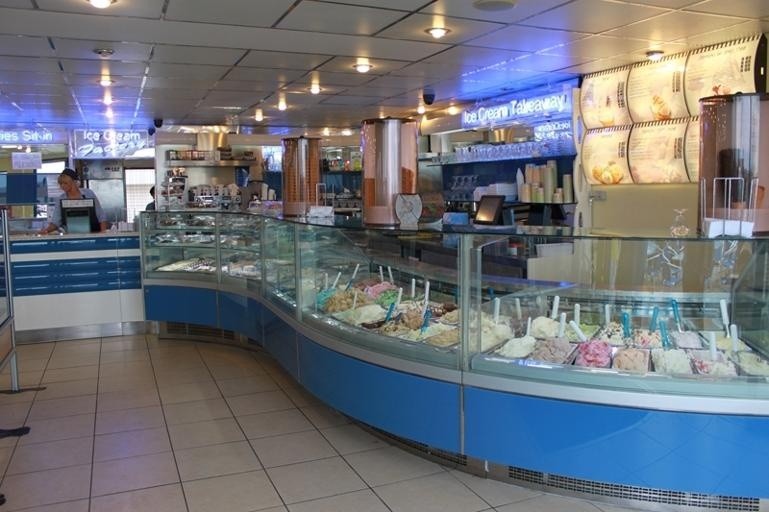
[353,65,372,73]
[425,27,450,39]
[648,52,664,60]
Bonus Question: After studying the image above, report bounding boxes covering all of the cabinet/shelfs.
[151,226,264,253]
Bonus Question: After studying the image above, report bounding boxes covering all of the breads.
[648,94,672,121]
[592,162,624,185]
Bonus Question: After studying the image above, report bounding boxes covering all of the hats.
[63,168,79,180]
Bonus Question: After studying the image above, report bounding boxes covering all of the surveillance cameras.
[153,118,164,128]
[148,127,155,135]
[423,89,436,105]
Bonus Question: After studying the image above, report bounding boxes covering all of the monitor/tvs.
[527,204,567,226]
[60,199,100,232]
[473,194,506,224]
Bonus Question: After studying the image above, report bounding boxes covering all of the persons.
[36,169,108,235]
[146,184,155,228]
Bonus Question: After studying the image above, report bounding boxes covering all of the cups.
[520,160,574,203]
[431,139,534,164]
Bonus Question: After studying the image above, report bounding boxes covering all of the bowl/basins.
[487,182,518,202]
